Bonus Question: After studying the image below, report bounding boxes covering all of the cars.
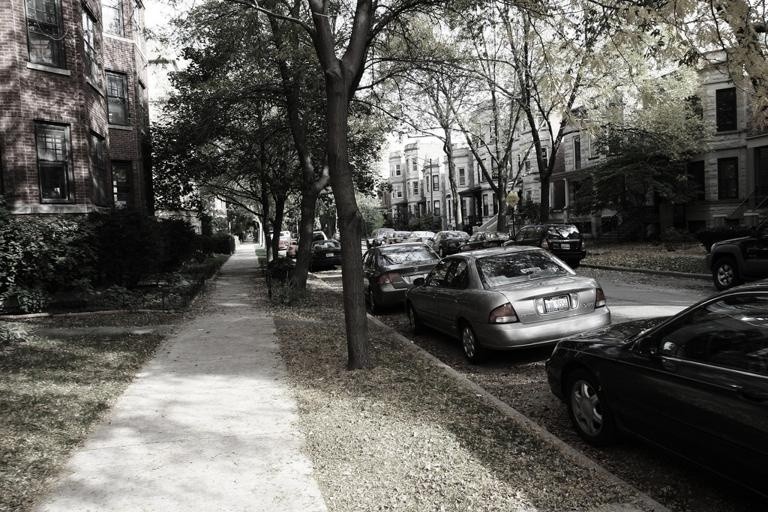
[279,231,342,272]
[709,218,768,292]
[545,277,768,511]
[363,223,610,365]
